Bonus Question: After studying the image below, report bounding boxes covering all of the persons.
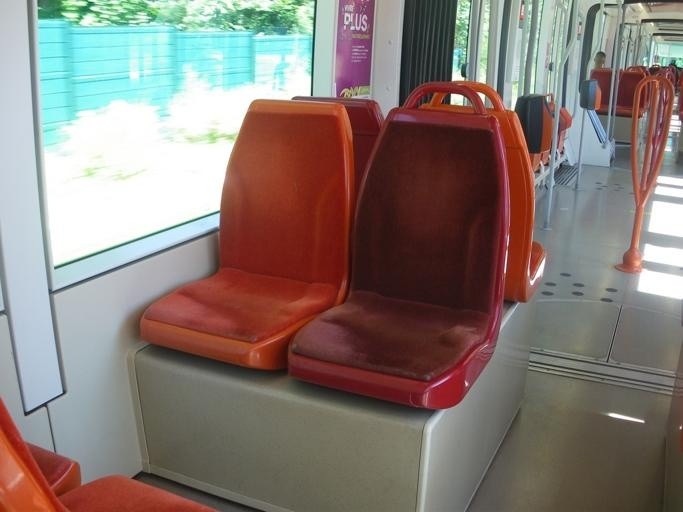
[593,50,607,69]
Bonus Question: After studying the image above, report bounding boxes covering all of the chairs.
[138,83,355,377]
[0,391,224,510]
[591,65,683,119]
[285,80,513,409]
[418,81,544,308]
